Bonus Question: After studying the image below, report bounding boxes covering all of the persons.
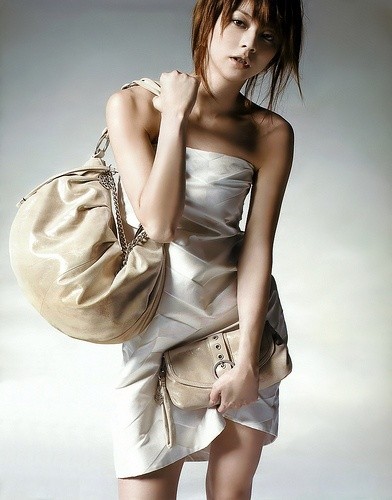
[105,1,305,500]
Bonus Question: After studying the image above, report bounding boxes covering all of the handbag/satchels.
[154,320,292,449]
[10,77,166,345]
